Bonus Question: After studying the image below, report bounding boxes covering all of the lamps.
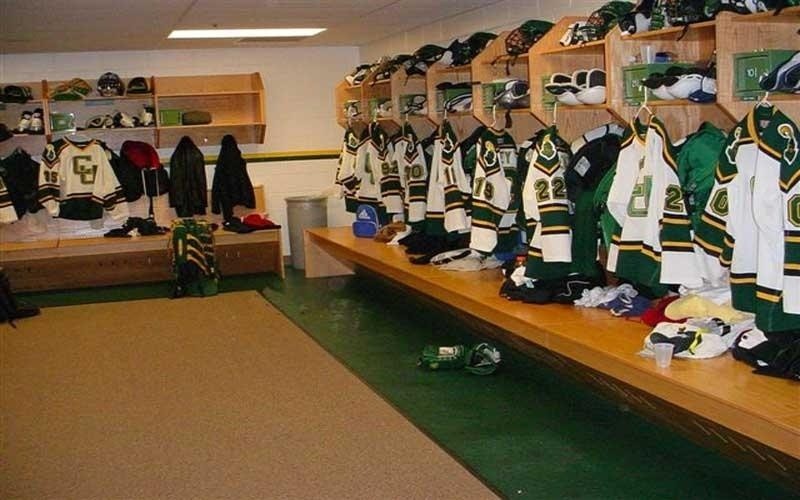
[167,29,326,39]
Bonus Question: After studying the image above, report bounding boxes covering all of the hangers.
[632,79,656,119]
[402,104,414,125]
[66,115,91,143]
[549,98,562,131]
[370,109,380,122]
[442,101,453,125]
[755,74,774,112]
[344,107,359,127]
[487,100,505,133]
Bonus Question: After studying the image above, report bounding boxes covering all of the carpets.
[258,261,799,500]
[2,273,260,309]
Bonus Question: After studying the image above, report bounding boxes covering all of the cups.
[655,343,675,368]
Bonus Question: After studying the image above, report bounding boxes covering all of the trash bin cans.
[283,195,328,270]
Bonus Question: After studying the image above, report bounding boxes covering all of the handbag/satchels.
[352,203,394,238]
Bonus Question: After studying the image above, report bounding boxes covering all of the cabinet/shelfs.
[0,186,287,296]
[302,225,800,492]
[0,71,268,156]
[335,7,800,140]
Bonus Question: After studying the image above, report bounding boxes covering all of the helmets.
[495,81,530,107]
[545,74,582,106]
[376,100,392,117]
[445,92,474,113]
[380,54,413,74]
[664,65,715,102]
[759,51,800,93]
[96,73,123,97]
[409,98,427,114]
[582,1,633,40]
[406,45,444,74]
[348,102,363,120]
[506,20,555,53]
[562,69,605,104]
[644,67,681,100]
[453,32,496,67]
[347,64,381,84]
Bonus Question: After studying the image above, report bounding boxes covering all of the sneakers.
[30,108,44,133]
[16,112,31,134]
